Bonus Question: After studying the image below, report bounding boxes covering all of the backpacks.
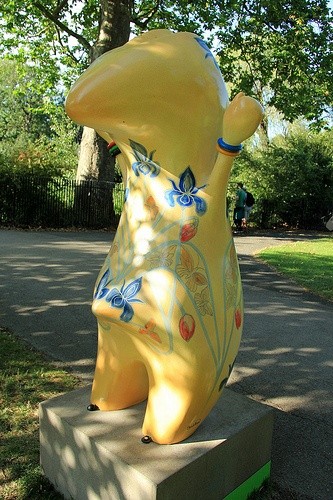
[245,192,255,207]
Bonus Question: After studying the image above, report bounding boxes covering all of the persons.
[231,183,246,234]
[242,188,253,227]
